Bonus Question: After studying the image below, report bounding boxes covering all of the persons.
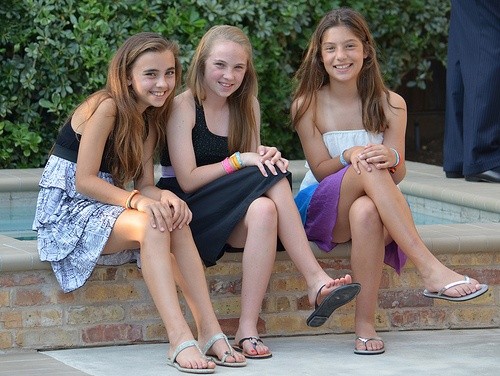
[443,0,500,183]
[156,25,361,359]
[32,32,247,373]
[291,9,489,355]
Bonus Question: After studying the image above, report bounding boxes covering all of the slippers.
[423,275,489,301]
[232,336,272,359]
[354,337,385,354]
[167,340,215,373]
[306,283,361,328]
[202,332,246,367]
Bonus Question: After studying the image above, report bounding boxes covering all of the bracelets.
[222,151,243,175]
[340,148,350,167]
[126,190,140,210]
[389,147,403,173]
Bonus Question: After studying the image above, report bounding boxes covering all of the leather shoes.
[446,165,500,183]
